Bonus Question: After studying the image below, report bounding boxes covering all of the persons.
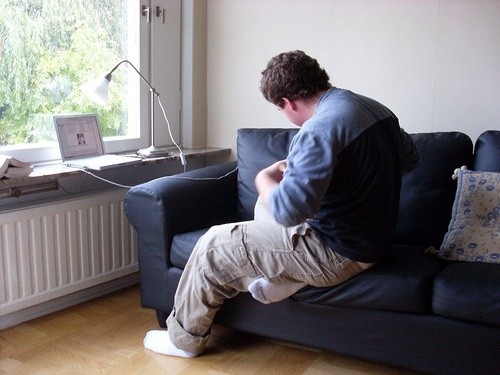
[142,50,422,359]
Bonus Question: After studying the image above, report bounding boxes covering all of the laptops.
[53,114,142,171]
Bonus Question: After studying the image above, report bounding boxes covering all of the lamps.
[79,60,188,173]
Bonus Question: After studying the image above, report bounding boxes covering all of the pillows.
[440,170,500,263]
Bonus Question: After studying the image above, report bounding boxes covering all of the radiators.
[0,187,139,317]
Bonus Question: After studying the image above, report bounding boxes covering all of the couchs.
[122,127,500,374]
